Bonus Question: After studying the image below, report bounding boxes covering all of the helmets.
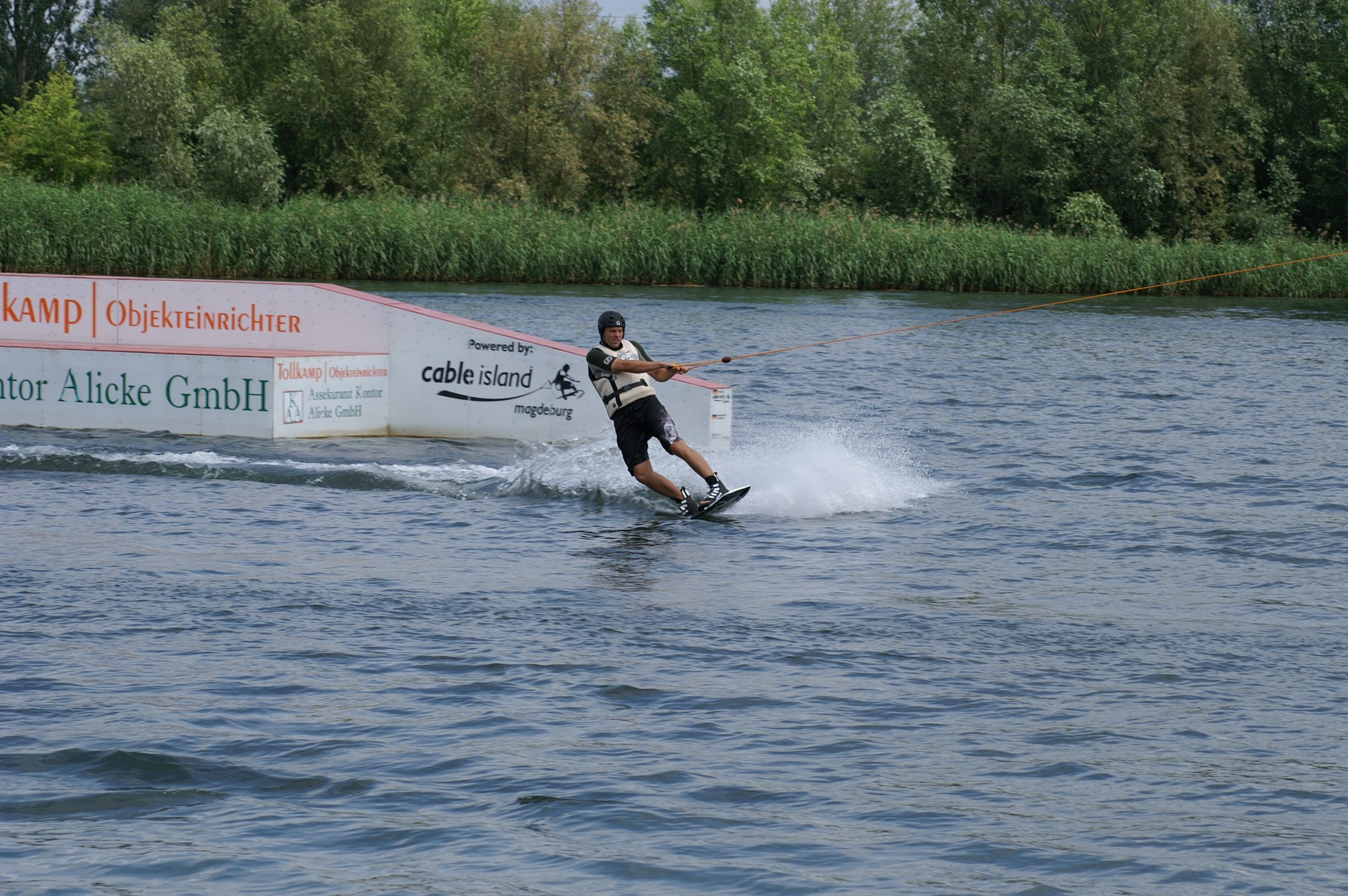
[597,311,626,336]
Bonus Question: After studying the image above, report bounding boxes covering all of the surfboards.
[690,485,751,521]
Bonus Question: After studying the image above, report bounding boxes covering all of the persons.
[586,310,729,515]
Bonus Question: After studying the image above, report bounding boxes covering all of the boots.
[700,472,729,506]
[673,486,699,518]
